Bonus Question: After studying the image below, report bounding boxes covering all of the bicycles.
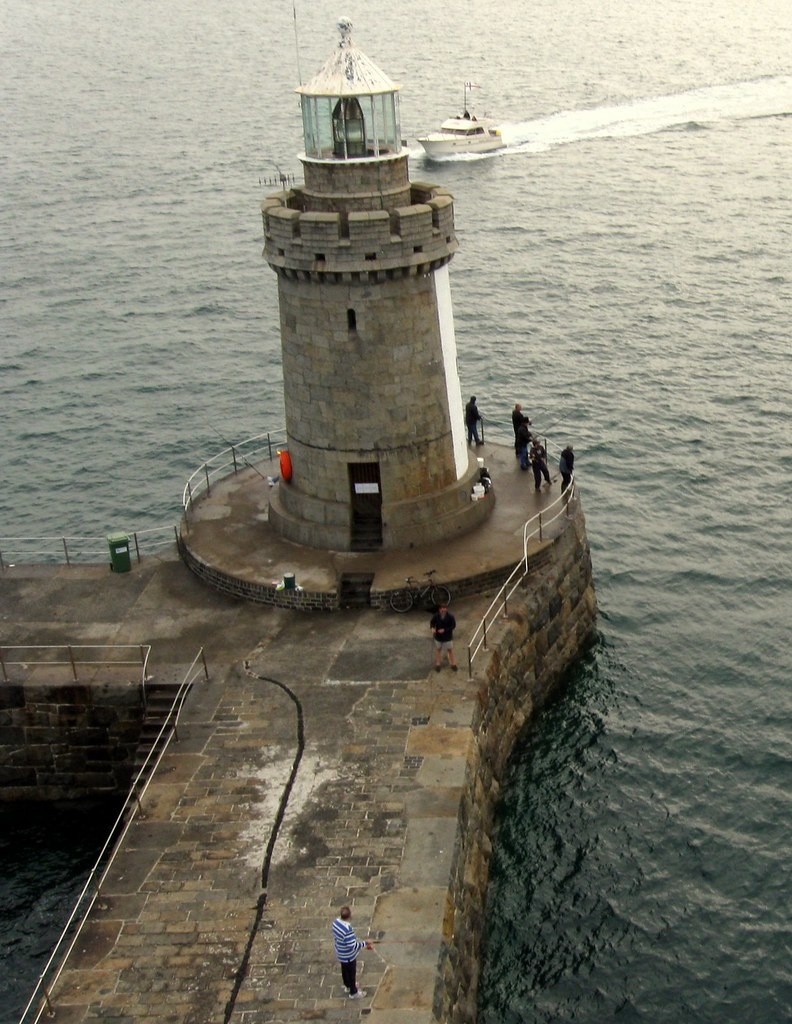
[388,569,453,614]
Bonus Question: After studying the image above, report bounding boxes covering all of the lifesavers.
[279,450,292,481]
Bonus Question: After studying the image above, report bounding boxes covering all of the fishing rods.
[186,405,265,479]
[531,397,595,440]
[543,440,612,486]
[529,392,574,425]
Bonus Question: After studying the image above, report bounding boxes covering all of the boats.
[416,81,508,156]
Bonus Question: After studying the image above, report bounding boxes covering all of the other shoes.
[547,480,552,485]
[476,441,485,445]
[343,982,360,993]
[521,464,529,471]
[527,462,532,466]
[534,488,541,493]
[434,665,441,672]
[349,989,370,1000]
[450,664,459,671]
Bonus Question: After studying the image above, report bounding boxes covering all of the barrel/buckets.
[472,485,485,498]
[476,457,485,468]
[470,494,479,501]
[283,573,296,589]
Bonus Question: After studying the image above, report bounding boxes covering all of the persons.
[331,906,374,1000]
[558,444,575,494]
[517,416,534,470]
[429,603,459,673]
[465,396,484,447]
[464,111,470,120]
[530,438,552,492]
[512,404,524,455]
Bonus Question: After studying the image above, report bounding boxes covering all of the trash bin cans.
[106,532,131,573]
